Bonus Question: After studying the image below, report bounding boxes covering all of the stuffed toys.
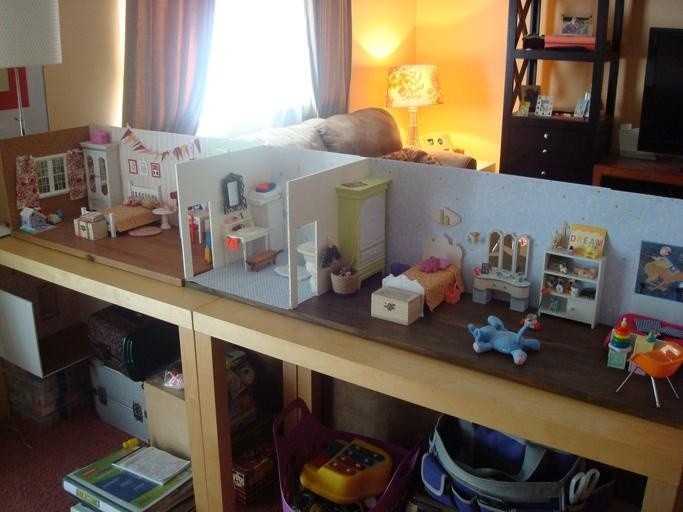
[468,312,542,365]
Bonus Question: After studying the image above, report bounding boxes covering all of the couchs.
[233,107,477,169]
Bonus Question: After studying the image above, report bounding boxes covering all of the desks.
[592,159,683,186]
[0,235,297,512]
[193,269,683,512]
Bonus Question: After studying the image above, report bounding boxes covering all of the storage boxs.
[87,359,150,444]
[144,349,254,460]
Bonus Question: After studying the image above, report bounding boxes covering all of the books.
[61,444,195,512]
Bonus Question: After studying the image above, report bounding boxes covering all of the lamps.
[386,63,443,147]
[0,0,63,136]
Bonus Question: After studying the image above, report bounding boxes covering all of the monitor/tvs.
[637,27,683,155]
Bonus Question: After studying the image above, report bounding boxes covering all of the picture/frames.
[535,95,554,116]
[557,12,594,37]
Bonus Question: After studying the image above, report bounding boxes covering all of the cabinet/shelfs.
[499,0,624,184]
[476,160,496,172]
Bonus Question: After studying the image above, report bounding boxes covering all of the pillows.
[315,107,403,158]
[232,122,328,151]
